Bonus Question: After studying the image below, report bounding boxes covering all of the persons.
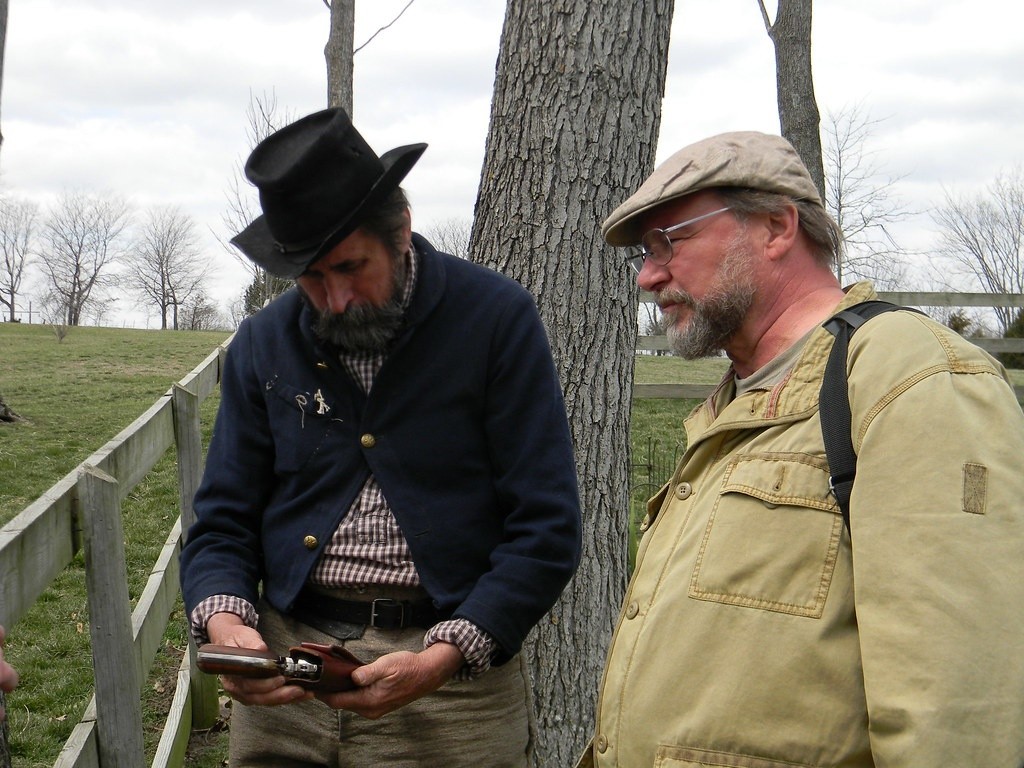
[594,130,1024,768]
[177,106,583,768]
[0,625,19,719]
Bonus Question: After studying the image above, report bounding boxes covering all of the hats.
[601,131,824,247]
[230,107,428,279]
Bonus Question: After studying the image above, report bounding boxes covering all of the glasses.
[623,207,731,274]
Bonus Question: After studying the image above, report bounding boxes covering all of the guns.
[194,640,370,693]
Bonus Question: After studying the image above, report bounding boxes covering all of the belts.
[295,586,454,629]
